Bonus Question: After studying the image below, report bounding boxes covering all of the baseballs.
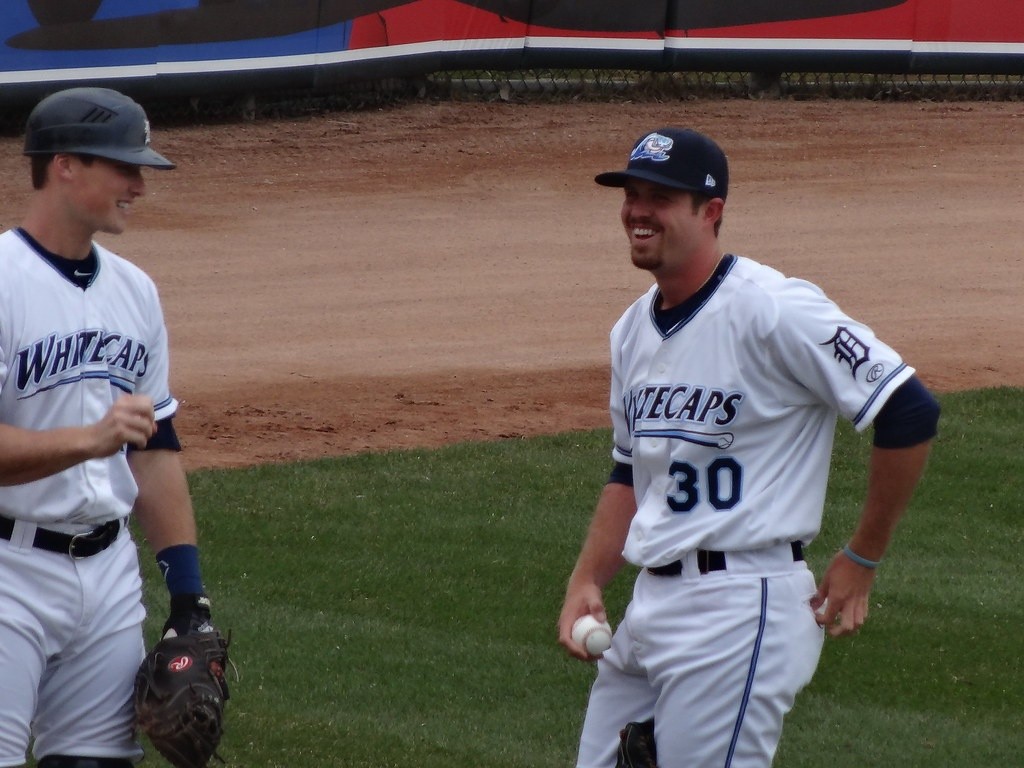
[571,613,613,658]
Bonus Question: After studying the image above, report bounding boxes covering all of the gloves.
[160,592,213,640]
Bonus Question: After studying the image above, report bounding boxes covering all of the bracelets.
[842,544,880,570]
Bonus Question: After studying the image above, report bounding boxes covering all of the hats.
[594,128,728,200]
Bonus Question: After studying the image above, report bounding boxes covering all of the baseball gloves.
[132,628,232,768]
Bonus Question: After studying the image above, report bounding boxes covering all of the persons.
[558,128,942,768]
[0,87,237,768]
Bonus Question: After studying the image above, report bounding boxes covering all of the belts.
[647,539,804,576]
[0,514,129,559]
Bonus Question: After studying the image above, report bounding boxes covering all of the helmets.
[23,87,177,171]
